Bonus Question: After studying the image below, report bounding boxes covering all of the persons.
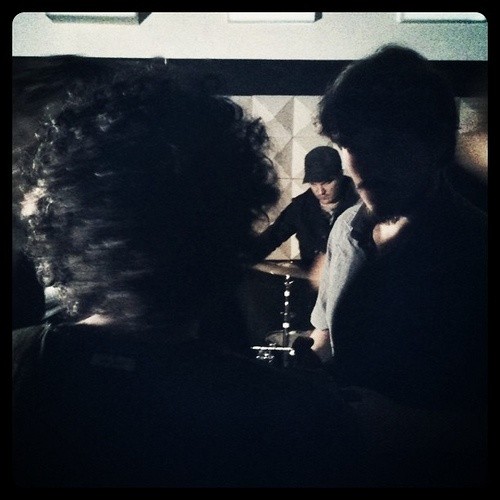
[308,45,491,453]
[8,70,346,489]
[251,146,358,271]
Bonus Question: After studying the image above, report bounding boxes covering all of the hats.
[303,147,342,182]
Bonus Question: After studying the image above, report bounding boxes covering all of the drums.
[249,346,296,368]
[267,330,312,347]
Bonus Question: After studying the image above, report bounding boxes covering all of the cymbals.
[244,260,312,280]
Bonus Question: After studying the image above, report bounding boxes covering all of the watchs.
[405,407,428,448]
